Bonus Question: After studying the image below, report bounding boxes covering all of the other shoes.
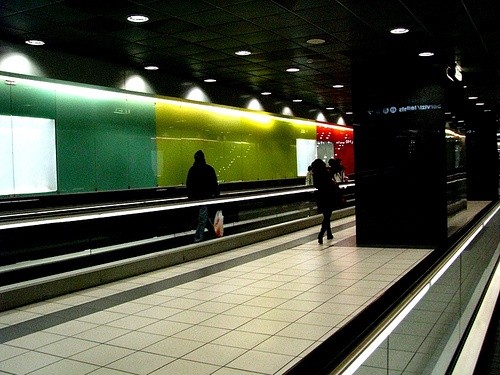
[327,235,333,239]
[317,235,323,244]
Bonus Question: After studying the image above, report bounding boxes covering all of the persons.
[188,151,220,247]
[306,158,351,244]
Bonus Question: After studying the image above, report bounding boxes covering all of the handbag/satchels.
[214,210,224,236]
